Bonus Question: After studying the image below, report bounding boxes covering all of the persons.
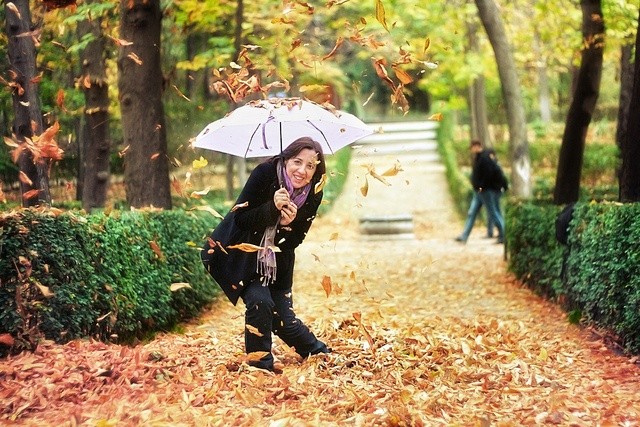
[486,150,507,237]
[199,136,344,371]
[454,140,507,244]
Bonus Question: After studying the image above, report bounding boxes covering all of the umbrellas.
[188,95,377,216]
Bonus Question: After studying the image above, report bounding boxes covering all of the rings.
[291,212,295,216]
